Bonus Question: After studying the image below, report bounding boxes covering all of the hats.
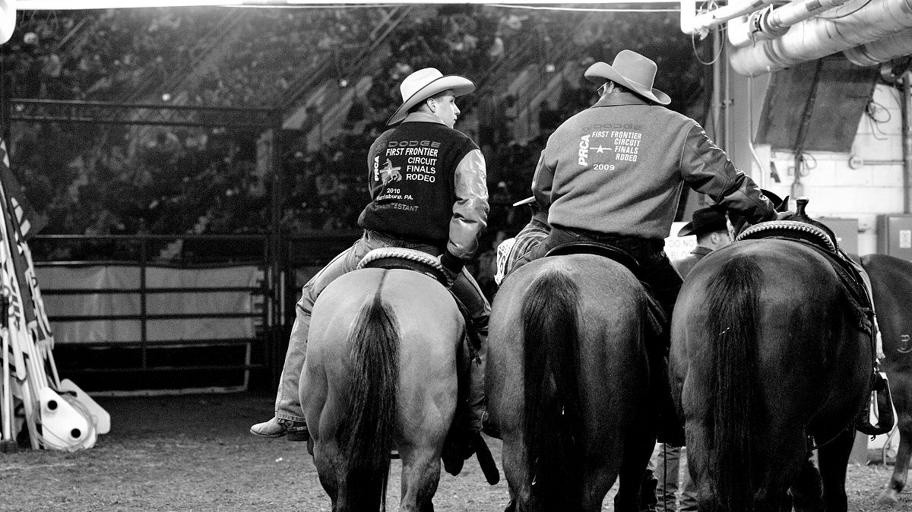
[678,203,724,237]
[385,67,476,128]
[584,49,671,105]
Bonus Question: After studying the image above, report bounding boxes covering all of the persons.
[249,66,493,439]
[504,48,797,444]
[2,4,716,272]
[652,206,734,512]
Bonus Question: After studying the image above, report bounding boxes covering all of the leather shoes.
[250,418,310,442]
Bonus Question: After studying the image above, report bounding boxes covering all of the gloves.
[437,252,462,284]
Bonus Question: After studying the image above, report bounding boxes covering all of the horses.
[299,266,471,512]
[668,232,869,512]
[482,250,666,512]
[845,250,912,507]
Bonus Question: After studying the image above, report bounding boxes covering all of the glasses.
[597,82,609,96]
